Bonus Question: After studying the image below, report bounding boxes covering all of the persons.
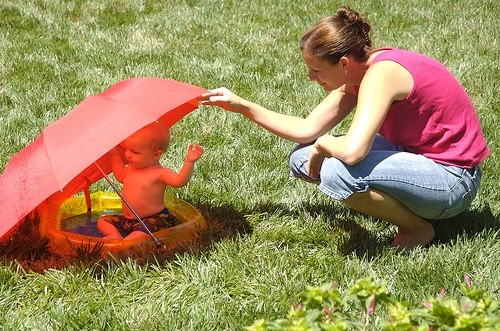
[194,6,490,254]
[97,120,205,242]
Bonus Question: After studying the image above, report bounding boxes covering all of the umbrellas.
[0,76,217,252]
